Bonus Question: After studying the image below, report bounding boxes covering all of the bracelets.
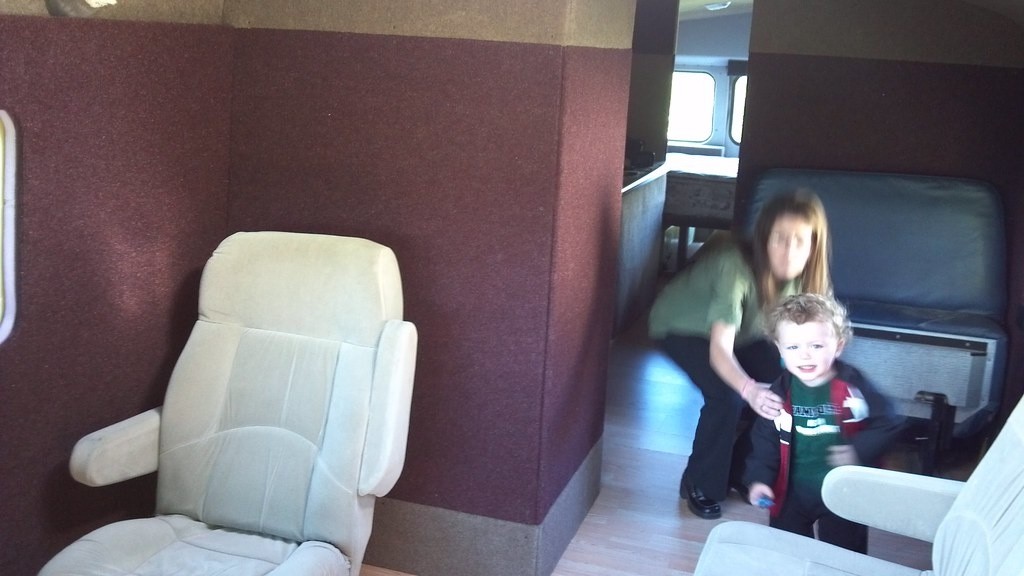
[740,378,755,399]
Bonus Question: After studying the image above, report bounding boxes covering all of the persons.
[743,283,906,555]
[648,186,832,519]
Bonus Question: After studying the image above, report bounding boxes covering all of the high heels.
[729,473,751,505]
[680,467,721,520]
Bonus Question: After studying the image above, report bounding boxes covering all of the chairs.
[693,394,1024,576]
[37,232,418,576]
